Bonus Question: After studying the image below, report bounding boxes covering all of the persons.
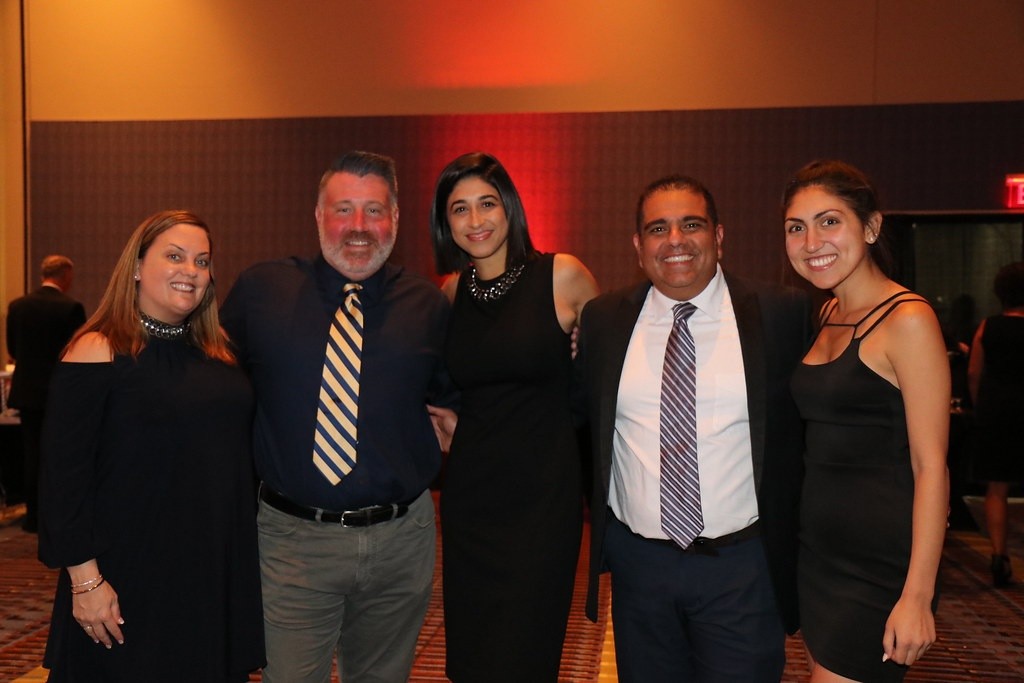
[6,255,87,531]
[28,150,952,683]
[965,263,1024,588]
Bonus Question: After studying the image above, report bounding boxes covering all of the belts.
[259,486,421,528]
[660,526,759,553]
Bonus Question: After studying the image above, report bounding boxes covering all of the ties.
[312,279,364,487]
[659,302,704,550]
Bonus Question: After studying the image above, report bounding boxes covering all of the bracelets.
[70,574,102,587]
[71,579,105,593]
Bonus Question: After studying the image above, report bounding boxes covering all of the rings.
[84,626,93,634]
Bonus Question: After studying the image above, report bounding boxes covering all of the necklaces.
[137,311,192,340]
[467,262,525,303]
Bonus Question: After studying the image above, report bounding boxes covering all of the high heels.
[991,554,1011,585]
